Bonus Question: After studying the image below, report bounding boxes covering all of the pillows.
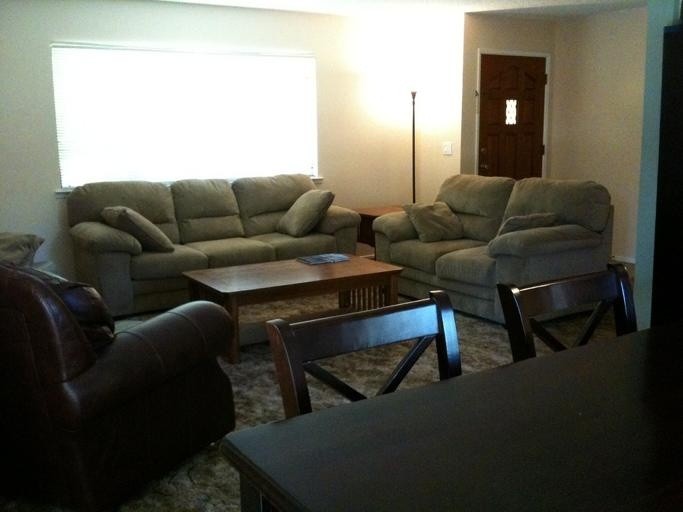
[102,206,176,254]
[494,212,557,240]
[277,188,336,238]
[400,202,465,243]
[0,230,45,268]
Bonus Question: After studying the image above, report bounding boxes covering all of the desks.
[221,319,683,512]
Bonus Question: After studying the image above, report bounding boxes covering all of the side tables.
[356,205,405,259]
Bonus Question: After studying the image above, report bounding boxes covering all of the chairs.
[497,262,638,363]
[0,259,237,512]
[264,289,463,418]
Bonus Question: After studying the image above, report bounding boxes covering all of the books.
[296,252,350,265]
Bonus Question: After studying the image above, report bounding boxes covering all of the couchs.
[372,173,615,332]
[68,174,362,320]
